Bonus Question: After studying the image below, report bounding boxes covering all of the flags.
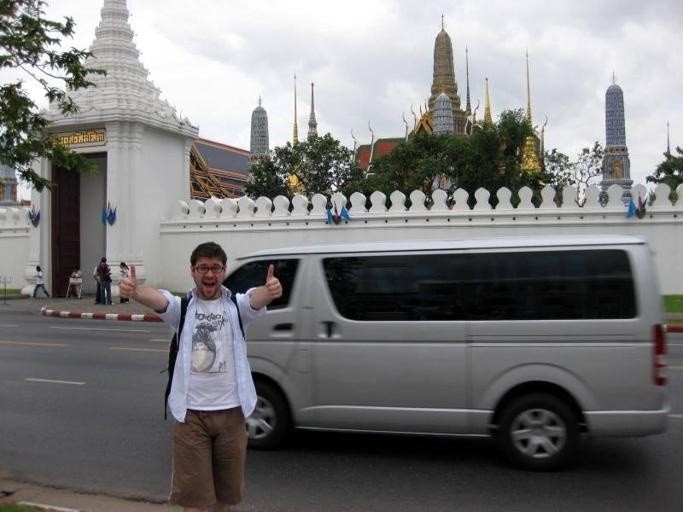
[28,204,40,228]
[625,193,649,218]
[326,200,352,224]
[102,201,117,225]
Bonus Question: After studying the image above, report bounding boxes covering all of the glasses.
[194,265,225,273]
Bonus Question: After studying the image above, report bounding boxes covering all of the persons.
[32,266,50,298]
[118,242,283,512]
[93,260,101,304]
[119,262,129,304]
[97,257,115,305]
[71,267,82,297]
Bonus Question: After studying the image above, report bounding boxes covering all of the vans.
[218,234,672,469]
